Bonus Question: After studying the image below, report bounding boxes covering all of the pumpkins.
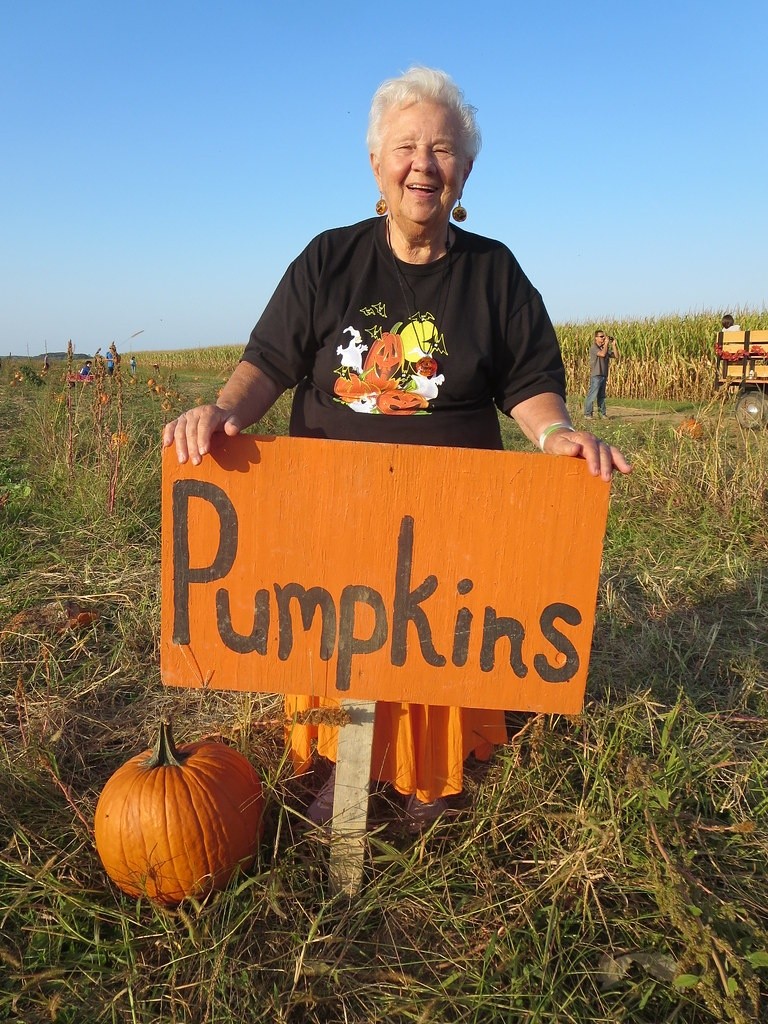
[94,720,264,904]
[10,371,230,446]
[683,415,701,430]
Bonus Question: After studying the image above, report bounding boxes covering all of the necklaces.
[387,214,452,377]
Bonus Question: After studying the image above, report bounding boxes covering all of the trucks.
[711,329,768,431]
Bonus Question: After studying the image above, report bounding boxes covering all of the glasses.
[597,336,604,338]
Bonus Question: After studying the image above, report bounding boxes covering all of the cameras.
[608,336,614,341]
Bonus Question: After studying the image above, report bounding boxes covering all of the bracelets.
[539,422,576,453]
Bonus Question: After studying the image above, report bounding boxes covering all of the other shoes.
[406,791,448,834]
[585,415,593,421]
[307,765,334,831]
[600,414,608,420]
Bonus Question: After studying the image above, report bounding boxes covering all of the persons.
[131,356,138,375]
[80,360,92,375]
[105,346,114,376]
[160,62,636,849]
[584,329,620,422]
[721,314,741,332]
